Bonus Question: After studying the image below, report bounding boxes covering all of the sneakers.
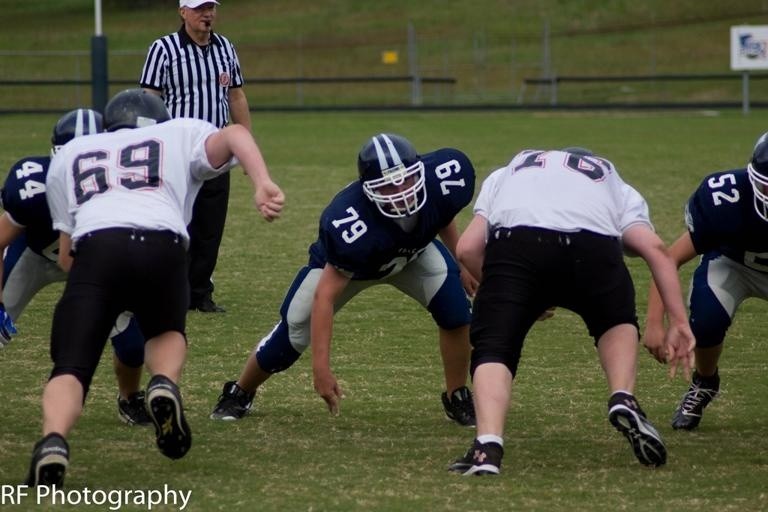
[143,375,191,459]
[448,440,503,476]
[117,391,151,425]
[608,391,667,466]
[22,432,70,489]
[440,386,477,428]
[208,380,256,422]
[671,368,720,430]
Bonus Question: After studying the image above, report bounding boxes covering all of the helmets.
[102,88,171,133]
[358,132,428,218]
[49,108,103,159]
[746,131,767,223]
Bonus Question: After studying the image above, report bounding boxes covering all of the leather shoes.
[190,299,225,312]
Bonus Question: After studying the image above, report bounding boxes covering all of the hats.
[179,1,221,10]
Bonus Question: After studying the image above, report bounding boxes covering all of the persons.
[138,0,254,315]
[21,85,287,491]
[637,128,768,433]
[1,106,157,427]
[205,131,478,430]
[446,144,700,481]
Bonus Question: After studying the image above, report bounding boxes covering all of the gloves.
[0,299,18,348]
